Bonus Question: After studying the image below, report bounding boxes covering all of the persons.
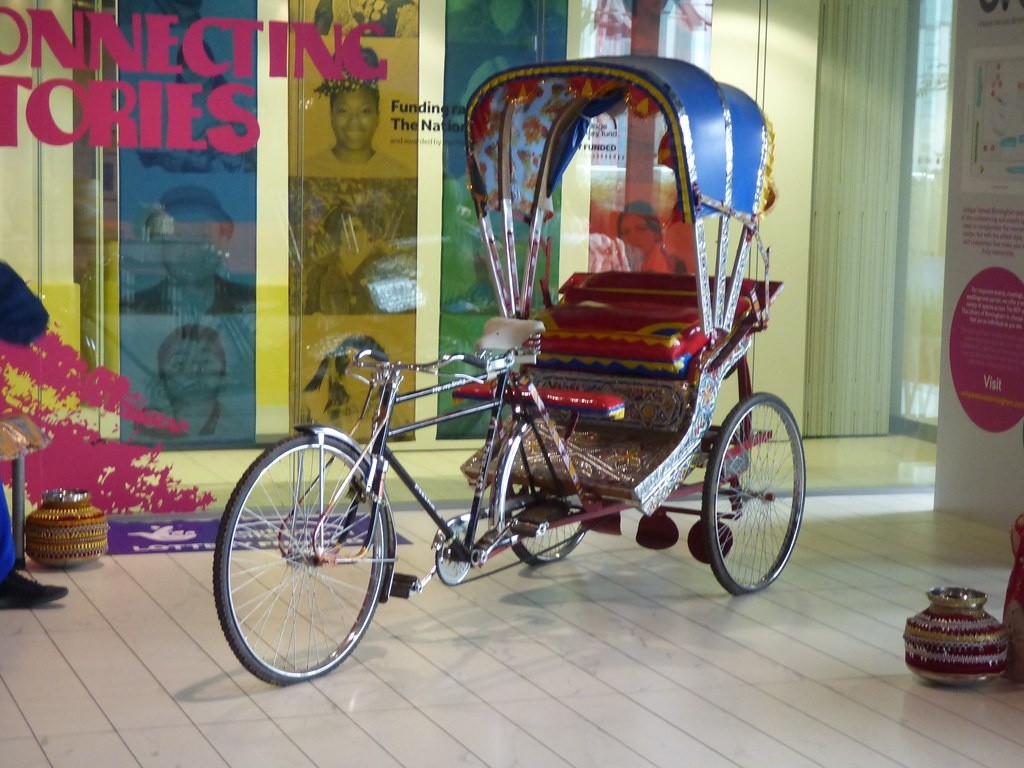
[0,262,69,611]
[304,195,417,442]
[120,185,256,313]
[618,200,688,274]
[303,45,416,178]
[130,324,254,434]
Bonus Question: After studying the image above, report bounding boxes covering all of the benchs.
[525,270,784,435]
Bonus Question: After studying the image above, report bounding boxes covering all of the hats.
[140,185,235,243]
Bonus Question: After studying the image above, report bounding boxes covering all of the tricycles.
[209,49,807,689]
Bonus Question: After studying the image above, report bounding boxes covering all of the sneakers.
[0,572,70,609]
